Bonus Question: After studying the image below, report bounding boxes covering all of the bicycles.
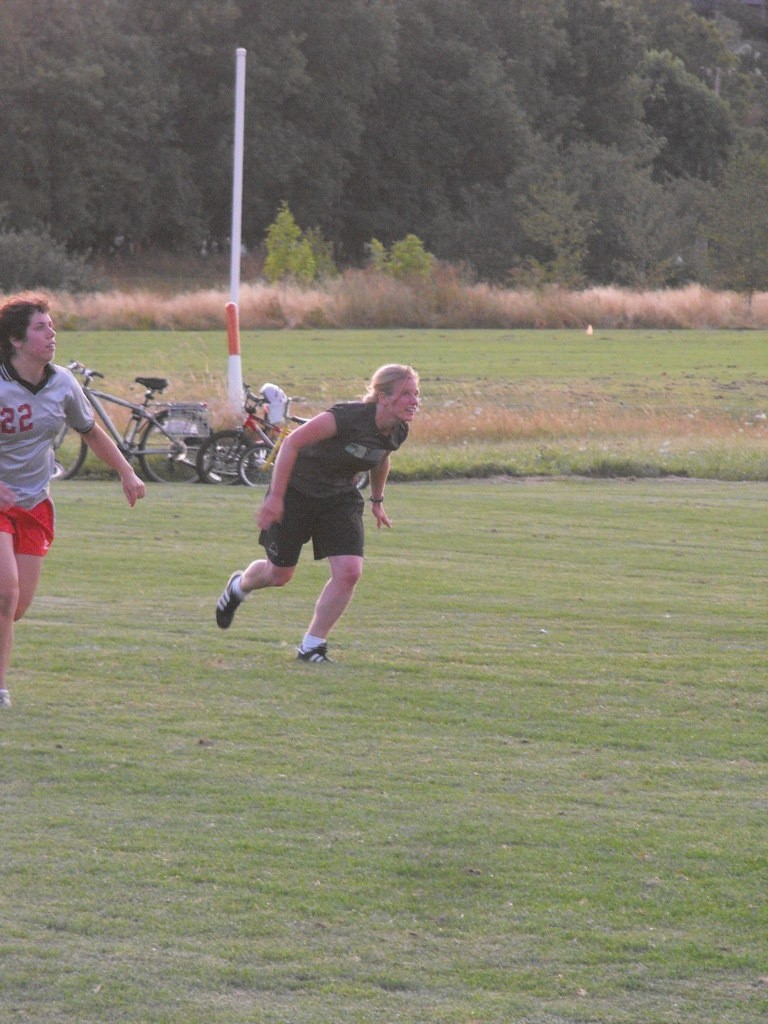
[53,358,217,485]
[196,381,371,491]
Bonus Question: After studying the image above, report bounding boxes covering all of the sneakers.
[296,644,335,665]
[216,570,243,629]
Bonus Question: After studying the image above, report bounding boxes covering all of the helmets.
[259,383,287,406]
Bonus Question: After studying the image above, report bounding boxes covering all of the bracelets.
[369,496,384,502]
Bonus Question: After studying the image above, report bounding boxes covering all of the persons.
[217,364,420,663]
[0,297,145,707]
